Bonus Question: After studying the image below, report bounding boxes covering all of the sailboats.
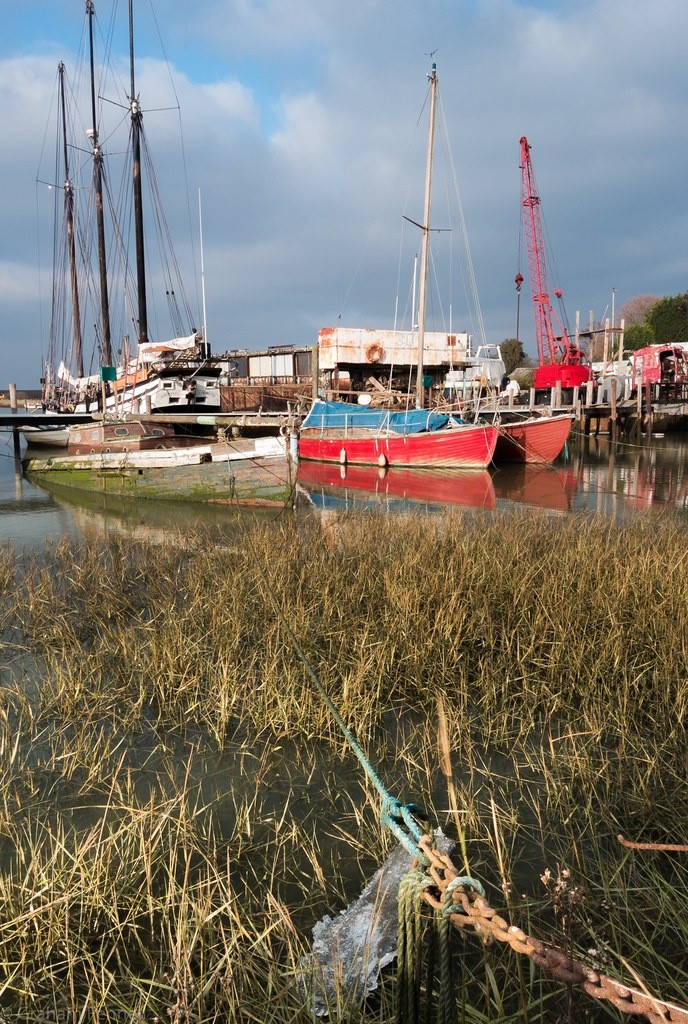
[17,0,240,447]
[290,58,504,469]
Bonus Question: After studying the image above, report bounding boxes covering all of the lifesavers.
[351,377,364,390]
[366,343,383,363]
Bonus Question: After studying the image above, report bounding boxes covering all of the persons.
[501,377,520,405]
[188,382,196,404]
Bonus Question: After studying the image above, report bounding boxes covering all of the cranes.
[513,136,598,389]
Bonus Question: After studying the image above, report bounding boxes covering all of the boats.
[289,458,499,522]
[18,470,322,564]
[485,464,573,517]
[489,410,579,465]
[22,399,302,508]
